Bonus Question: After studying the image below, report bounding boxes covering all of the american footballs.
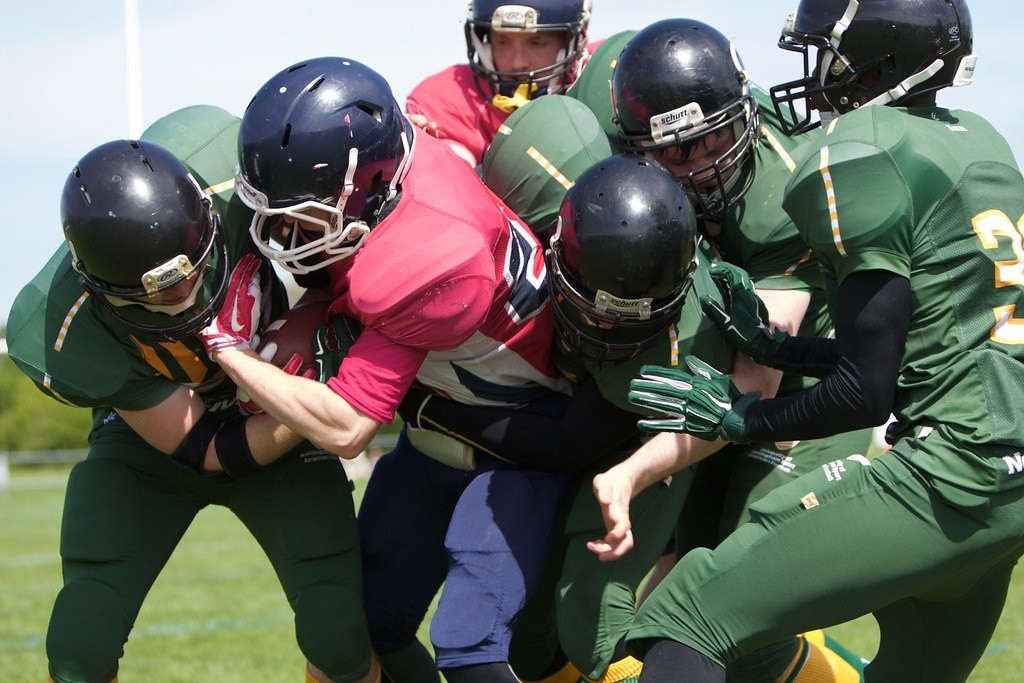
[254,298,338,373]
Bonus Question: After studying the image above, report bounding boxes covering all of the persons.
[6,104,383,683]
[201,0,1024,683]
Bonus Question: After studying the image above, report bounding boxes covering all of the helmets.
[607,18,761,152]
[58,138,219,299]
[544,153,696,320]
[466,0,588,61]
[237,56,414,233]
[794,1,976,108]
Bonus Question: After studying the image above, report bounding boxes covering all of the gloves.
[192,253,268,363]
[700,259,804,377]
[234,317,328,421]
[627,354,763,453]
